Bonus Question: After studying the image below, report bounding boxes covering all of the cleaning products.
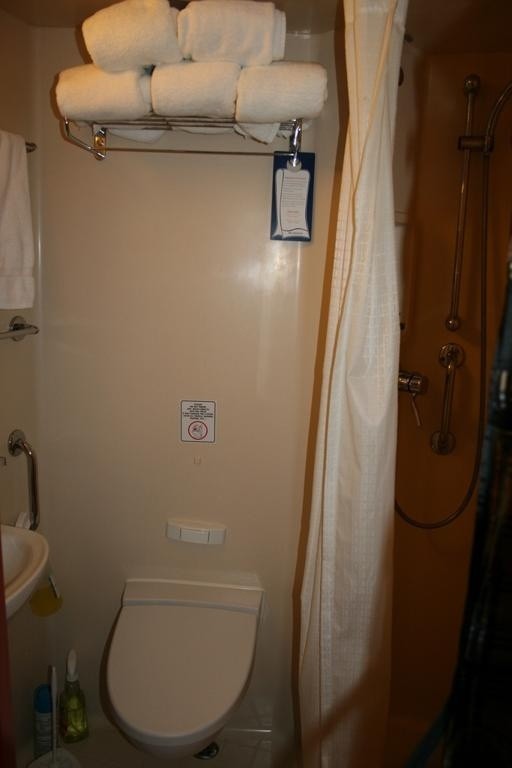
[34,686,52,757]
[59,649,88,744]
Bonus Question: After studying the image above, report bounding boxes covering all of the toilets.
[106,578,263,760]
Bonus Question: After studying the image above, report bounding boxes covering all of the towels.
[0,131,36,313]
[56,2,330,144]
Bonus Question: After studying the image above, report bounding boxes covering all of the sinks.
[0,524,50,621]
